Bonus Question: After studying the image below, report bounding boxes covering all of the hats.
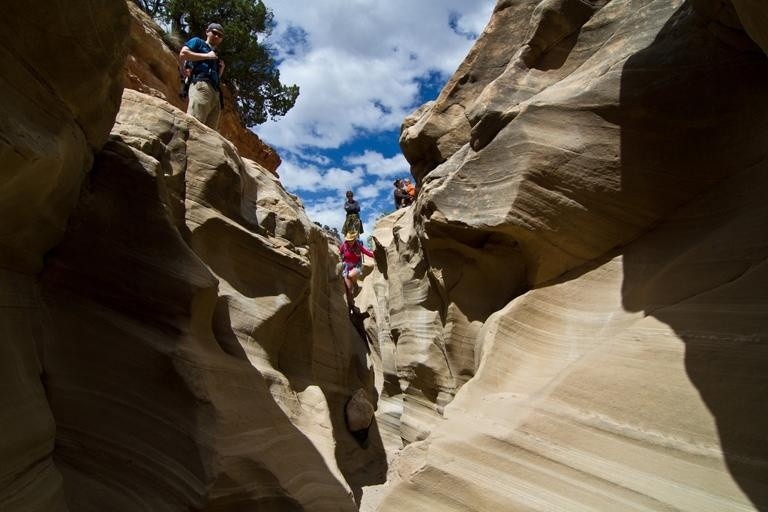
[345,230,359,241]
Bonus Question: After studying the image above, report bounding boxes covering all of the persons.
[402,175,416,202]
[335,230,377,312]
[178,23,226,133]
[393,178,410,208]
[343,190,362,261]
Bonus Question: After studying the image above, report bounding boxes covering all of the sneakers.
[351,286,359,297]
